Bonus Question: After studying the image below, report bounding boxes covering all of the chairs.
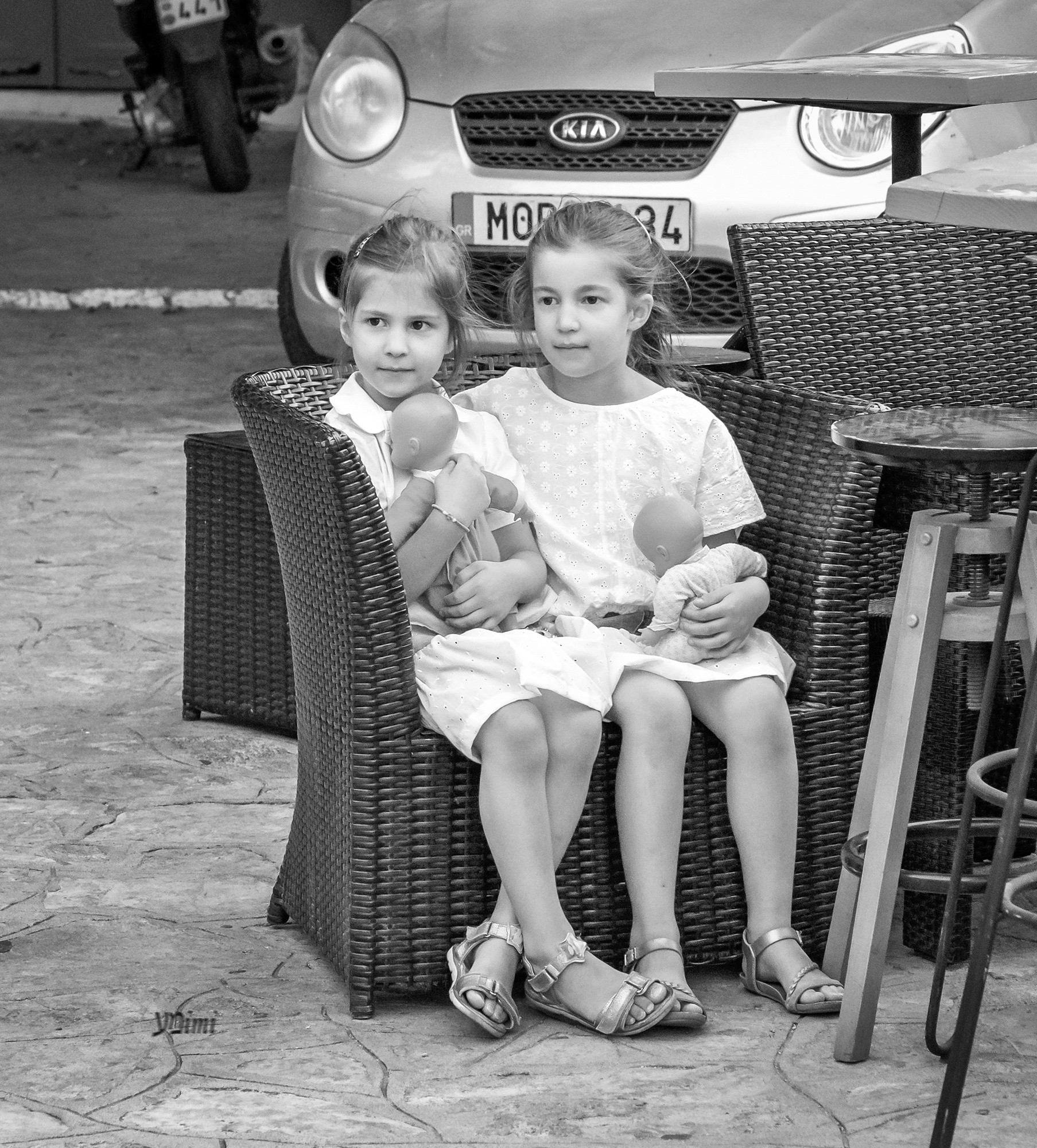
[181,221,1037,1013]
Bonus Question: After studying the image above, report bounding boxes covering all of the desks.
[655,54,1037,184]
[885,139,1037,232]
[822,410,1037,1148]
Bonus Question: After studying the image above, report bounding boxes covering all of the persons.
[378,395,535,640]
[447,201,844,1029]
[593,493,770,672]
[310,213,680,1040]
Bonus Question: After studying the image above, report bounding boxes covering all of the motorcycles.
[114,0,298,193]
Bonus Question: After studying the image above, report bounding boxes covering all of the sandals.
[742,926,845,1011]
[445,918,525,1036]
[527,935,679,1033]
[623,940,708,1027]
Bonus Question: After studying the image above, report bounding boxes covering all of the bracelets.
[431,502,470,532]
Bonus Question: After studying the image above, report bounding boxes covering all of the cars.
[279,0,1036,368]
